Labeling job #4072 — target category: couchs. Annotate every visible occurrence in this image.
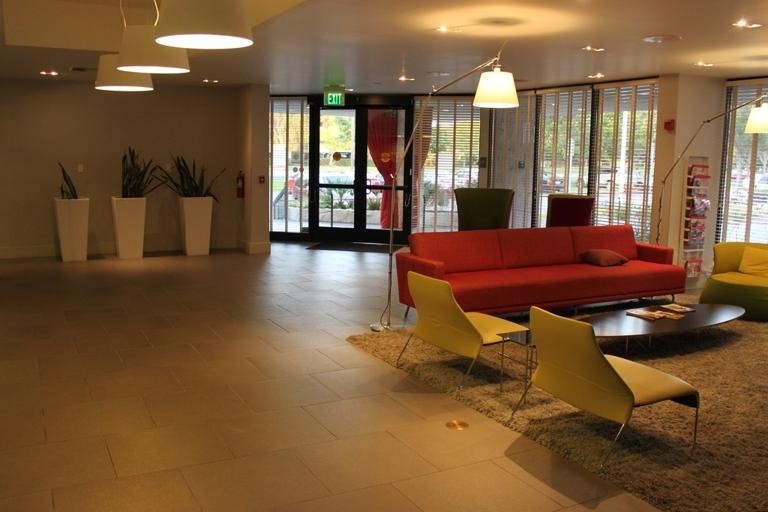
[396,224,686,322]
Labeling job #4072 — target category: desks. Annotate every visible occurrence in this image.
[496,330,535,404]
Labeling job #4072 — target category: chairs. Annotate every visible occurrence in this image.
[396,272,530,401]
[509,305,700,469]
[454,188,515,232]
[699,242,768,323]
[547,194,595,227]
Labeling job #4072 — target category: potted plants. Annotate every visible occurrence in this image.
[150,150,227,256]
[52,161,90,262]
[110,144,168,259]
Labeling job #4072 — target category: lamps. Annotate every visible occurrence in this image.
[370,58,519,332]
[153,0,254,49]
[94,54,154,93]
[656,94,768,246]
[115,24,191,75]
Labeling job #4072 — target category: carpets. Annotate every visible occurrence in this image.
[306,243,404,253]
[346,295,768,512]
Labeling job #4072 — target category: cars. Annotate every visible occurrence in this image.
[286,170,384,203]
[576,165,620,191]
[625,174,653,193]
[540,170,566,192]
[425,168,479,204]
[751,171,768,203]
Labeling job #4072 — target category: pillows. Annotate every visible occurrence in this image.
[738,246,768,277]
[576,249,629,266]
